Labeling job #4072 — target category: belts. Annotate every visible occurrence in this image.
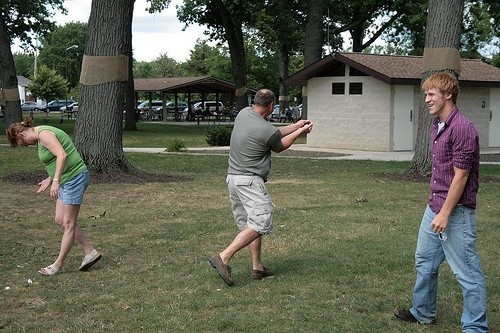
[457,204,463,206]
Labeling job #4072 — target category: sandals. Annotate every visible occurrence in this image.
[79,249,102,272]
[37,264,63,276]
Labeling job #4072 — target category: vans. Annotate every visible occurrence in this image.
[154,101,188,113]
[41,99,75,113]
[182,101,224,117]
[136,100,166,114]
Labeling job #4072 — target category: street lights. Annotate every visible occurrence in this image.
[65,45,78,78]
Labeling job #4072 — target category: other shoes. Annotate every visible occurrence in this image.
[395,309,436,325]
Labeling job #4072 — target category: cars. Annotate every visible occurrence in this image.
[123,100,145,114]
[264,103,303,124]
[59,102,78,113]
[21,101,41,112]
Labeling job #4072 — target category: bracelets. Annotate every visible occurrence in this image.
[53,179,59,181]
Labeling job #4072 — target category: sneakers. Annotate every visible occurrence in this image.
[208,252,234,287]
[251,266,273,280]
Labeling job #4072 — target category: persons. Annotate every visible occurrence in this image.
[395,74,489,333]
[7,117,102,276]
[208,89,314,286]
